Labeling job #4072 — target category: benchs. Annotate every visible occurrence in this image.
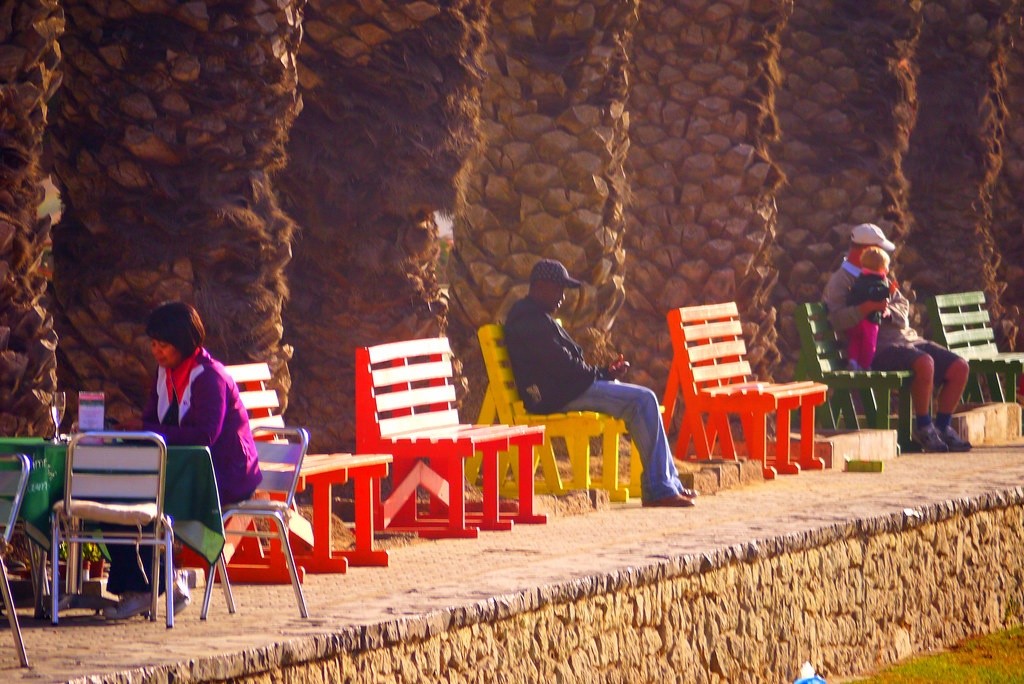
[465,323,665,503]
[173,362,393,586]
[787,301,922,455]
[926,290,1024,435]
[354,337,546,539]
[662,301,828,479]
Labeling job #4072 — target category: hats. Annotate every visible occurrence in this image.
[851,223,896,251]
[531,259,580,288]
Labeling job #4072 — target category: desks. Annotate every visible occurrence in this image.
[0,436,226,621]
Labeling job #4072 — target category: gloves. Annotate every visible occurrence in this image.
[607,354,630,381]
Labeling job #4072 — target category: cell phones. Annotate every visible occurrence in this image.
[617,362,630,371]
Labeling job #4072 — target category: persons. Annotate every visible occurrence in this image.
[826,223,971,454]
[104,303,264,620]
[504,259,698,508]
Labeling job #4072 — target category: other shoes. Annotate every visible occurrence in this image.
[642,495,695,506]
[847,359,873,373]
[682,489,697,498]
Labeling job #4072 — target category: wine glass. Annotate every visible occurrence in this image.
[51,391,66,445]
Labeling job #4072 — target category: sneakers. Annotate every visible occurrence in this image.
[913,425,948,452]
[173,573,191,614]
[940,425,971,450]
[104,593,151,618]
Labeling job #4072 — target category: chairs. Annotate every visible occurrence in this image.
[145,425,310,620]
[51,432,173,628]
[0,453,30,667]
